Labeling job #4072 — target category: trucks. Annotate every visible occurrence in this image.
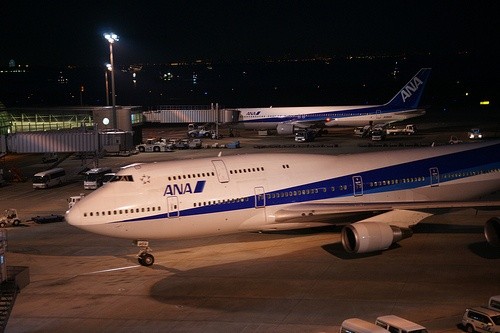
[135,137,176,153]
[354,125,414,141]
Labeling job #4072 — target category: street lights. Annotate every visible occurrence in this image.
[105,64,113,106]
[105,33,120,106]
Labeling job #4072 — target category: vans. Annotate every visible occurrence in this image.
[375,315,428,333]
[340,318,392,333]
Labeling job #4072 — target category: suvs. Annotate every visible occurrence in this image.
[454,295,500,333]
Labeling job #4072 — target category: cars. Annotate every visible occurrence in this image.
[294,132,307,143]
[468,129,484,142]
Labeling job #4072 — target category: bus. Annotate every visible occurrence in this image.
[83,167,112,191]
[32,168,66,190]
[102,173,117,186]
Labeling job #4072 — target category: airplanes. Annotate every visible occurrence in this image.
[234,68,432,143]
[65,140,500,266]
